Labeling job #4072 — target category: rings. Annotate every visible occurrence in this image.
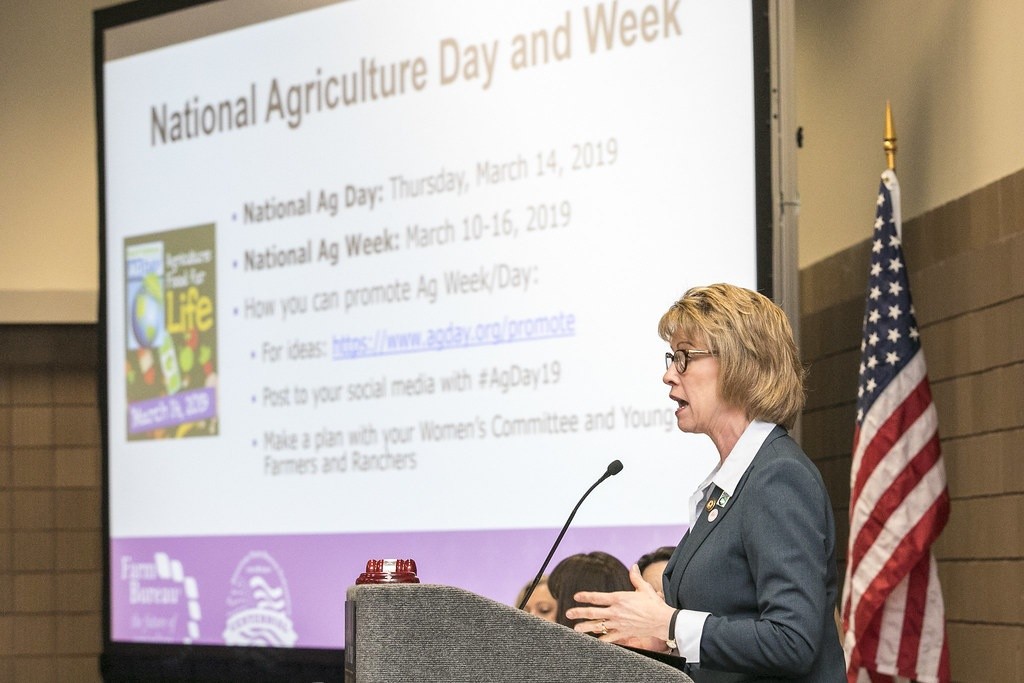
[595,618,611,636]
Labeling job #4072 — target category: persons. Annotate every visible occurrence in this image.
[514,544,679,639]
[566,285,851,683]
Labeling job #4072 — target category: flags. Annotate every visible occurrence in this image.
[832,166,957,683]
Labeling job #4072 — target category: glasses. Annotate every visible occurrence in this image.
[665,350,719,374]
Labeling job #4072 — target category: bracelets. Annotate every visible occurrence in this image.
[664,606,684,657]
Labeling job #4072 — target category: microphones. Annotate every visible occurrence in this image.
[519,460,624,610]
